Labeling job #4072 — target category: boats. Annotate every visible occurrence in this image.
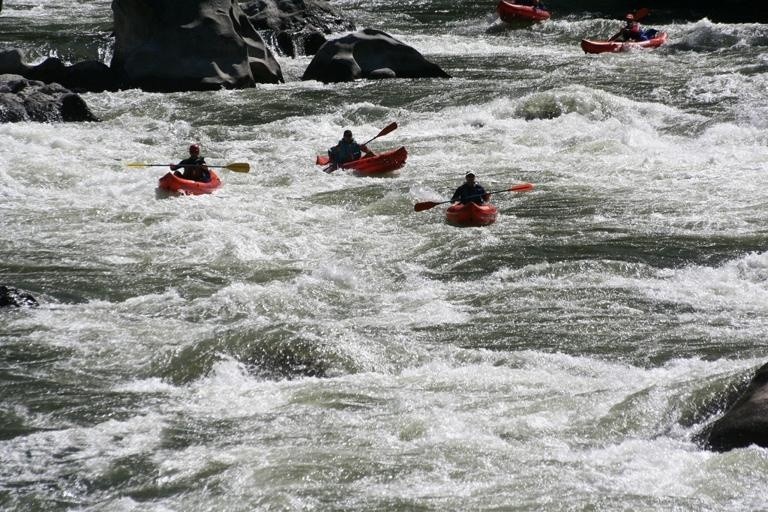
[314,145,409,176]
[447,200,498,228]
[581,32,668,54]
[497,0,551,27]
[157,167,222,196]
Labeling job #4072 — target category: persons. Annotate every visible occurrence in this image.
[618,14,644,43]
[168,144,211,182]
[329,130,377,162]
[450,169,492,207]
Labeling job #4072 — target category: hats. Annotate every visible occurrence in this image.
[466,170,476,177]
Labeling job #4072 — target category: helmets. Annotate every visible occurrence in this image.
[189,144,200,155]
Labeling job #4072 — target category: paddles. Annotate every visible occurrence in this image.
[414,184,532,211]
[127,161,249,172]
[323,122,397,173]
